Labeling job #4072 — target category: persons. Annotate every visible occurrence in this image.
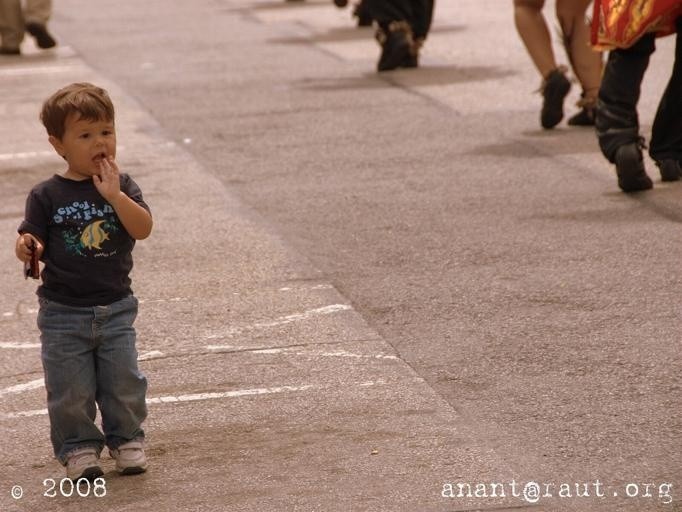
[0,0,57,55]
[593,1,682,192]
[512,1,605,128]
[361,0,436,72]
[15,82,153,483]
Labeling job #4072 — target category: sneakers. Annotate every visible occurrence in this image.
[376,20,421,71]
[108,441,148,474]
[66,448,104,483]
[0,48,20,55]
[657,158,682,182]
[568,109,598,126]
[541,69,570,129]
[25,22,55,49]
[615,144,653,191]
